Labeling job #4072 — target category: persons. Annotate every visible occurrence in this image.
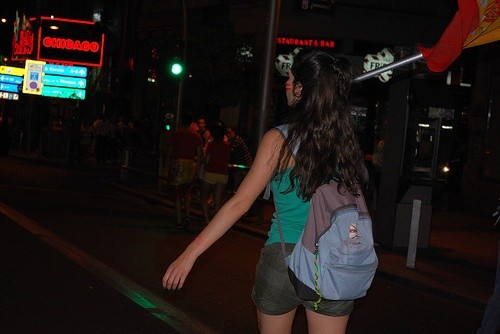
[165,115,255,230]
[162,48,371,334]
[368,139,384,192]
[50,108,152,168]
[405,124,430,188]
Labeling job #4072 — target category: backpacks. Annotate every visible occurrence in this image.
[274,124,379,300]
